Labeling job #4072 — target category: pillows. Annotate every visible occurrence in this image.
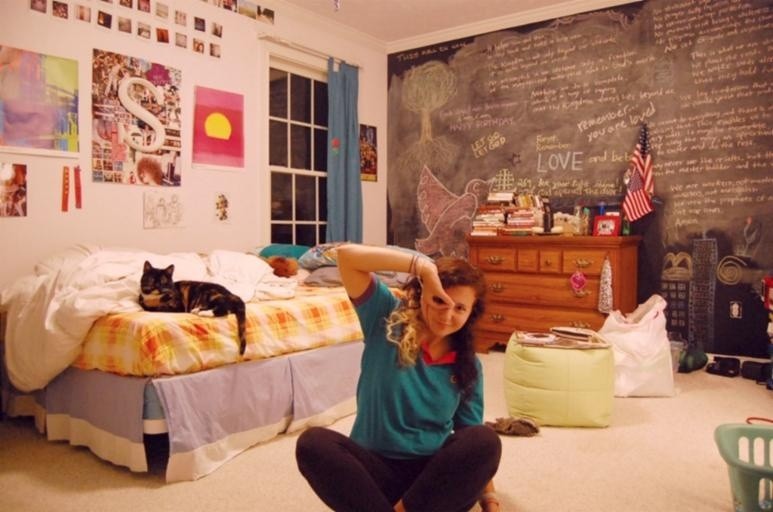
[304,267,395,287]
[252,243,312,262]
[296,241,398,276]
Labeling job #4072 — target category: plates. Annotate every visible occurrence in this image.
[523,332,555,342]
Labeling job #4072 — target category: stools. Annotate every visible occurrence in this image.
[501,324,616,433]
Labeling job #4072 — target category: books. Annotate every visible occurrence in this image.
[471,187,546,237]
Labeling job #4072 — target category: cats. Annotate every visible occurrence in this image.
[137,260,248,356]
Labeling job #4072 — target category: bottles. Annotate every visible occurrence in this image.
[573,206,593,235]
[540,191,552,232]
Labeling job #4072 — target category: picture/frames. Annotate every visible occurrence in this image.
[0,40,85,162]
[603,208,621,216]
[592,214,622,237]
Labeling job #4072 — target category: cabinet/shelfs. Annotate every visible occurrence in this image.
[461,230,643,356]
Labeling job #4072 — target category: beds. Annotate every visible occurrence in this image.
[0,239,437,480]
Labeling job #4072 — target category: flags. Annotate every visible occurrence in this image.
[621,118,655,222]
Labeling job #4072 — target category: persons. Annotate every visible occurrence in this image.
[296,243,501,511]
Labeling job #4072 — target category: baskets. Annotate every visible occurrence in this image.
[712,420,773,512]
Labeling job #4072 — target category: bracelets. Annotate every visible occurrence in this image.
[479,492,499,509]
[408,254,421,275]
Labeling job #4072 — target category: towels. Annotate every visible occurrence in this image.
[596,253,614,314]
